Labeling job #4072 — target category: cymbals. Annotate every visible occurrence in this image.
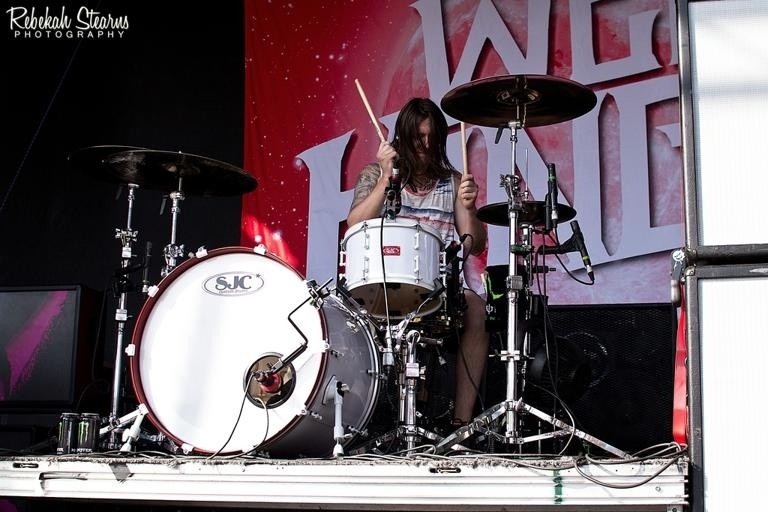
[68,144,257,195]
[442,76,596,128]
[478,201,576,226]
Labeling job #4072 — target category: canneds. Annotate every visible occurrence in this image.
[56,412,98,458]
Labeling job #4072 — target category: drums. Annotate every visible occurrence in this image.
[131,245,383,457]
[55,413,101,456]
[338,218,448,318]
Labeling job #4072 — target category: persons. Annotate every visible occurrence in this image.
[347,97,491,451]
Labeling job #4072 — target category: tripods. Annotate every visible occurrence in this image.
[98,169,179,456]
[345,316,479,456]
[423,119,638,462]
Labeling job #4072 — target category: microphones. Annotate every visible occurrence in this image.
[252,371,282,393]
[548,163,558,221]
[570,220,592,274]
[391,166,402,214]
